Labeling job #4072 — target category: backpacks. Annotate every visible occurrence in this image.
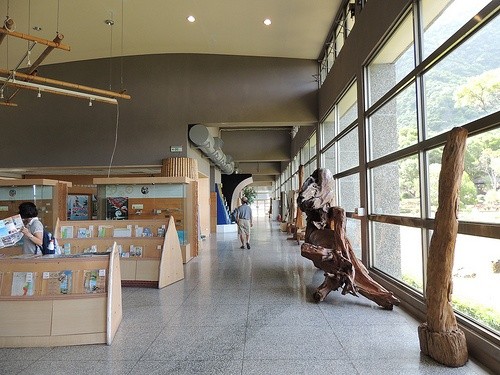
[30,220,56,255]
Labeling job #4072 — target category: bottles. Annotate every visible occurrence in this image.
[49,239,54,250]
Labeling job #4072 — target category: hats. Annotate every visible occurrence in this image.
[242,197,248,202]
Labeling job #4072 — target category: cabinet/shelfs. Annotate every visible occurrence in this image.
[0,178,199,347]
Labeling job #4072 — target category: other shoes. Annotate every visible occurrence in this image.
[240,245,245,249]
[246,243,250,249]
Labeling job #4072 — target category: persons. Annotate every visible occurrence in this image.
[236,197,253,250]
[18,202,55,255]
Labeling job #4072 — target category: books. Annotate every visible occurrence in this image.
[10,224,167,296]
